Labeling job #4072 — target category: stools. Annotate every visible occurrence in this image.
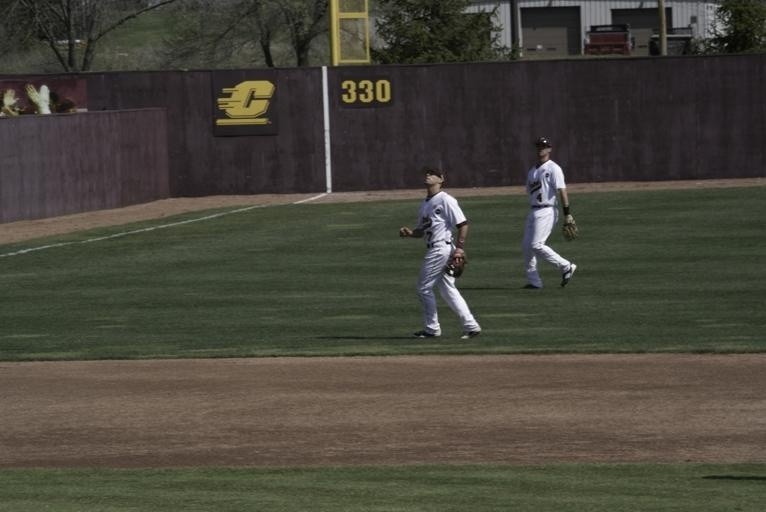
[427,241,450,248]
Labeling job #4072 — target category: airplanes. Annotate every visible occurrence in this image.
[562,213,579,241]
[444,248,466,278]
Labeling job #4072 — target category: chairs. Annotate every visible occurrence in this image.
[560,264,577,287]
[521,284,539,289]
[414,330,440,338]
[460,331,478,339]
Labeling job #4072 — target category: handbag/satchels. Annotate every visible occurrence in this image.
[563,206,570,215]
[456,237,466,249]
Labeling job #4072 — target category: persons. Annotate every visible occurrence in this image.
[1,82,80,117]
[522,136,577,289]
[399,165,482,340]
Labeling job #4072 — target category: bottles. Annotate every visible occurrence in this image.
[422,166,446,179]
[534,136,553,147]
